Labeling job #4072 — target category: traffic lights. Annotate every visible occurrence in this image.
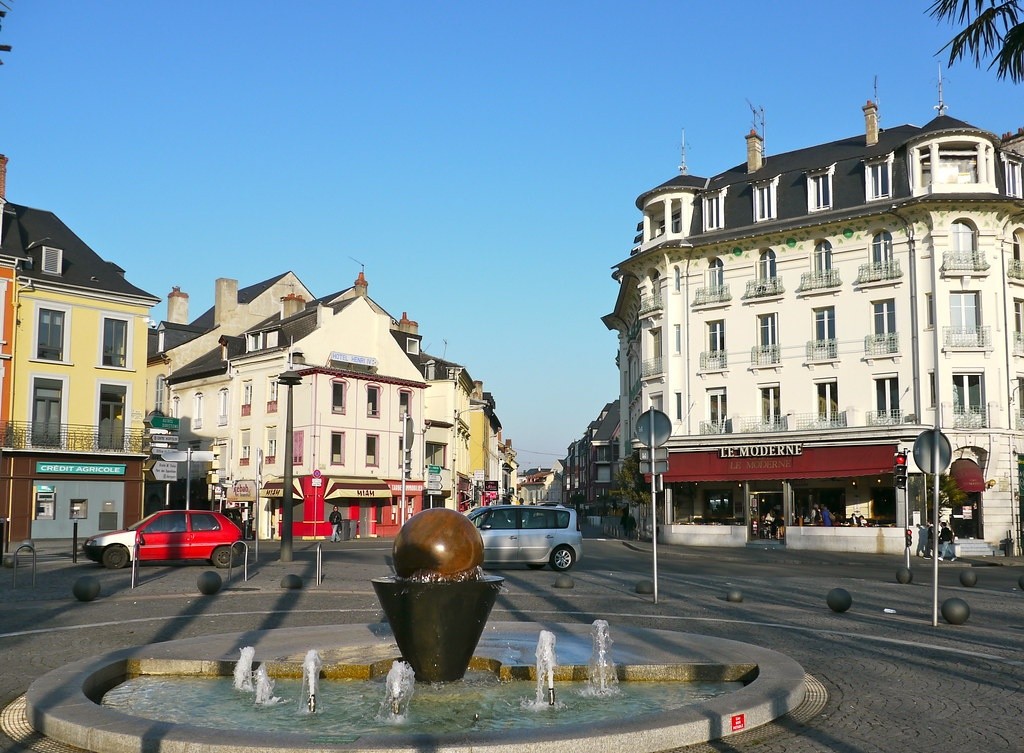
[907,529,912,548]
[894,454,907,489]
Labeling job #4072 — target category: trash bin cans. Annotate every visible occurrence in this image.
[338,519,350,541]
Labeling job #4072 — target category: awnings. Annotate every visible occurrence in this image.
[949,460,986,493]
[324,479,393,500]
[259,478,304,500]
[644,444,898,484]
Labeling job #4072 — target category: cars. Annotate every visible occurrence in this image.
[83,509,242,568]
[466,505,583,570]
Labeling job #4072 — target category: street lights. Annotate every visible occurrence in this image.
[452,404,485,510]
[278,372,302,560]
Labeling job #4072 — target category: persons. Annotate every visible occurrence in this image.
[626,513,636,541]
[765,514,772,539]
[850,513,860,525]
[329,505,342,543]
[622,503,629,516]
[612,503,618,515]
[939,522,957,561]
[813,504,821,523]
[923,519,942,559]
[821,504,831,526]
[620,512,629,536]
[859,515,867,523]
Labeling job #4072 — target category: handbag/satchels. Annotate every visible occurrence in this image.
[829,512,835,521]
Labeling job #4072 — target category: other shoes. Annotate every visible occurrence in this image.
[923,556,931,559]
[951,557,957,561]
[938,556,943,561]
